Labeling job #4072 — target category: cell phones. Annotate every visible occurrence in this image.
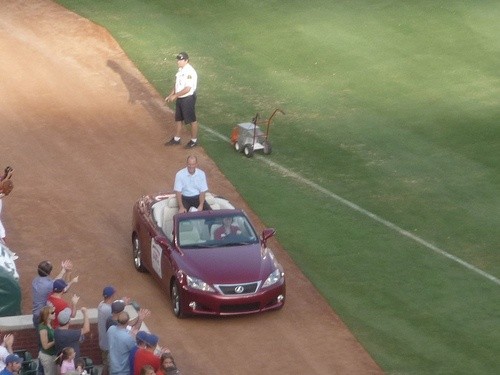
[4,166,13,180]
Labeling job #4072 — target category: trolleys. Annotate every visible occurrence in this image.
[232,107,285,158]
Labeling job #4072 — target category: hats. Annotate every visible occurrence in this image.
[53,279,67,292]
[103,287,116,297]
[57,308,71,324]
[138,333,158,346]
[6,355,20,363]
[112,300,126,312]
[177,52,189,60]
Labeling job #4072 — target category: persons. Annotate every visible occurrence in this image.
[37,306,56,375]
[54,307,89,369]
[32,259,79,375]
[0,329,23,375]
[105,300,180,375]
[214,217,243,242]
[97,286,130,368]
[55,347,83,375]
[174,155,215,235]
[163,51,197,149]
[44,279,80,329]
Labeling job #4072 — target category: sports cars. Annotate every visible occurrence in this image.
[129,192,287,318]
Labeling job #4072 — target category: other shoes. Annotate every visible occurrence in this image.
[165,137,183,145]
[185,140,198,149]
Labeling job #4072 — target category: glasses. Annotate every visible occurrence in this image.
[51,311,55,314]
